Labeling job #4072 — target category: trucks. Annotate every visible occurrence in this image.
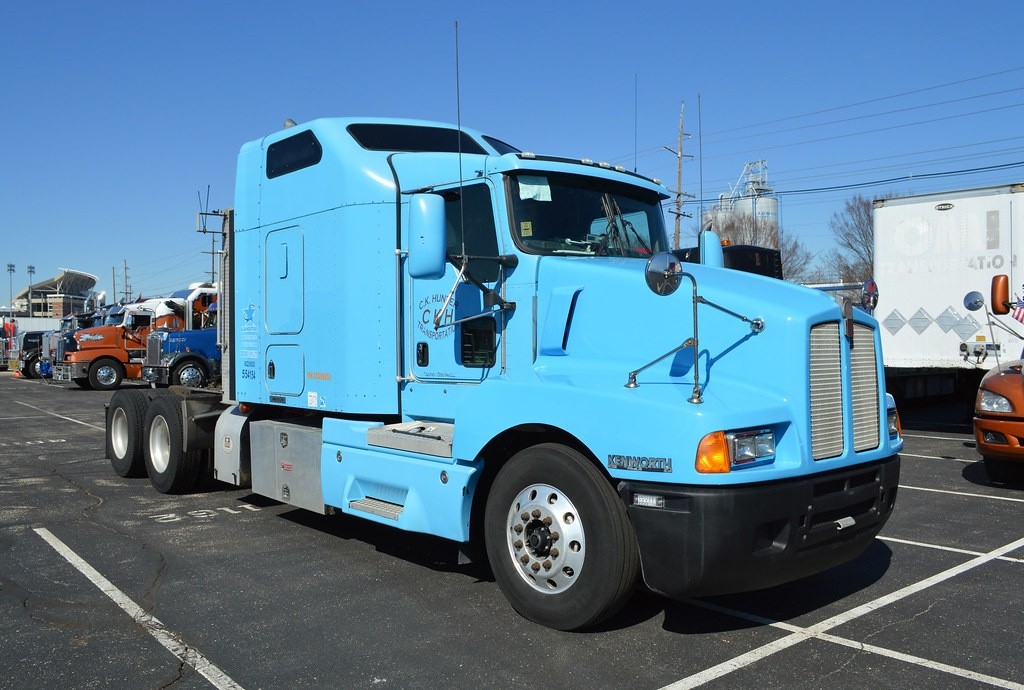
[140,298,224,390]
[871,182,1024,381]
[8,282,220,391]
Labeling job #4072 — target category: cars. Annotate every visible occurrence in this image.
[974,354,1023,481]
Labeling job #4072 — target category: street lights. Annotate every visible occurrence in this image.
[6,263,16,318]
[26,265,36,318]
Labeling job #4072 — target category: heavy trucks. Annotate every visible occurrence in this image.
[101,19,904,631]
